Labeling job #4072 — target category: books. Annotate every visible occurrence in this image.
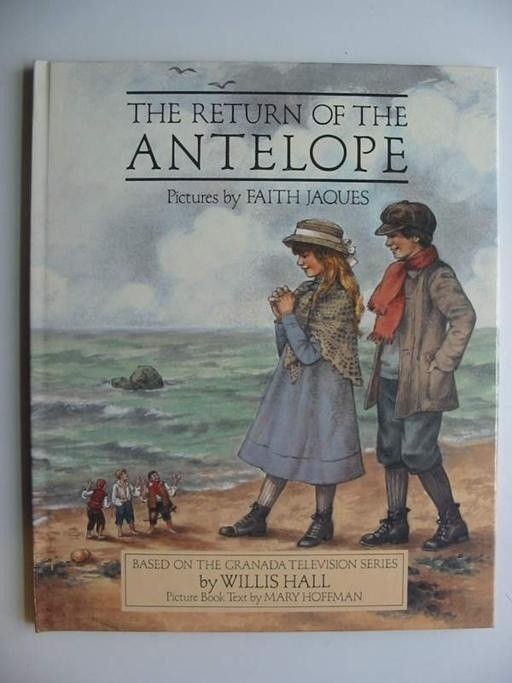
[18,50,503,636]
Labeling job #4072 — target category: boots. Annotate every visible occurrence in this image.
[295,505,336,548]
[218,502,271,538]
[421,504,471,550]
[359,507,411,548]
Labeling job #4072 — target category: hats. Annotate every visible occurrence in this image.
[372,201,439,243]
[281,217,357,261]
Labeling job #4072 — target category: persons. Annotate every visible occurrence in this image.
[217,218,366,550]
[81,477,113,541]
[142,469,182,537]
[110,466,142,538]
[360,199,479,555]
